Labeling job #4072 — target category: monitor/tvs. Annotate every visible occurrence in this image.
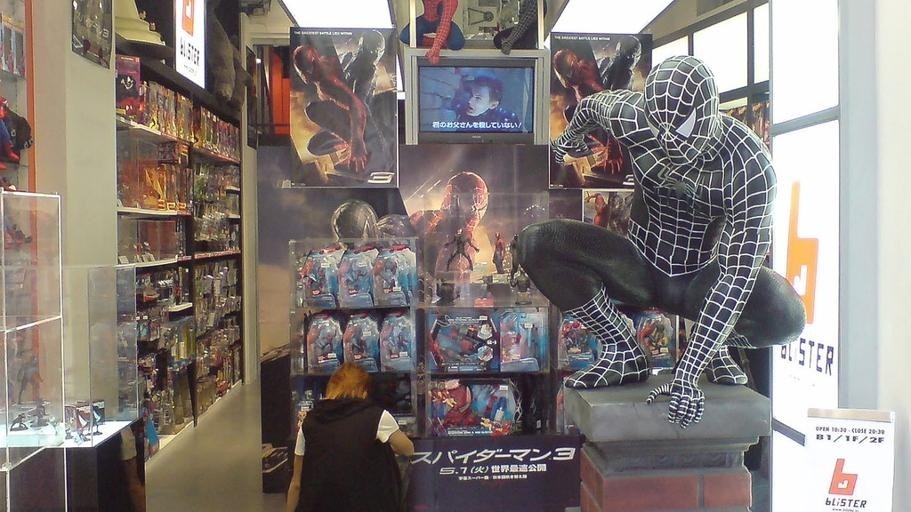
[413,55,536,143]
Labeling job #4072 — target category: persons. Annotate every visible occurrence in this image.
[492,231,506,274]
[595,35,644,93]
[292,44,370,175]
[508,232,525,280]
[510,267,539,306]
[442,228,484,273]
[410,170,491,289]
[334,30,396,176]
[607,192,637,236]
[444,74,522,132]
[330,198,424,262]
[399,0,464,66]
[584,192,610,228]
[553,48,625,178]
[284,362,415,512]
[510,56,806,428]
[0,96,106,444]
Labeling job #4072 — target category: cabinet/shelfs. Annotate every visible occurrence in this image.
[261,37,703,436]
